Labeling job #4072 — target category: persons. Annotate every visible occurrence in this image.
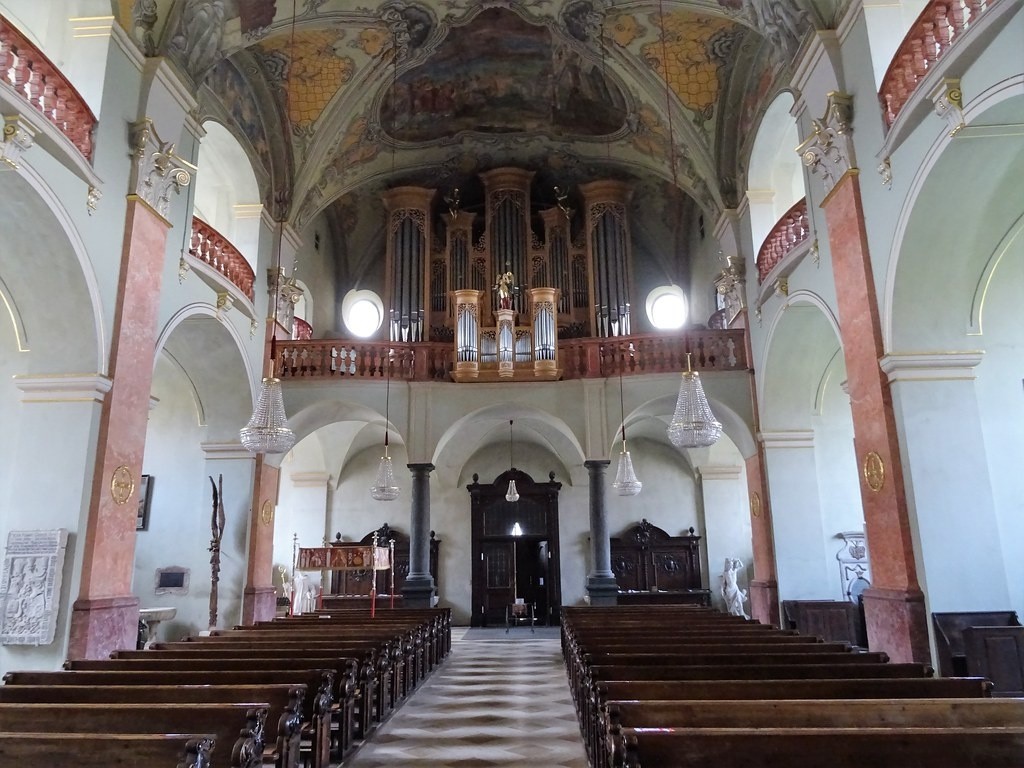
[282,570,318,615]
[719,557,750,622]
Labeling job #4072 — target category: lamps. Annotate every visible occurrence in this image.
[240,1,724,502]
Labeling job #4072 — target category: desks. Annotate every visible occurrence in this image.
[313,592,403,608]
[617,589,713,606]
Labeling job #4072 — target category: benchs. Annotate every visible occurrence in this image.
[0,600,1023,768]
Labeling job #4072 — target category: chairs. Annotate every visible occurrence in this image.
[505,603,537,633]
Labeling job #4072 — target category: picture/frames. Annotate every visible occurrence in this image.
[136,474,149,530]
